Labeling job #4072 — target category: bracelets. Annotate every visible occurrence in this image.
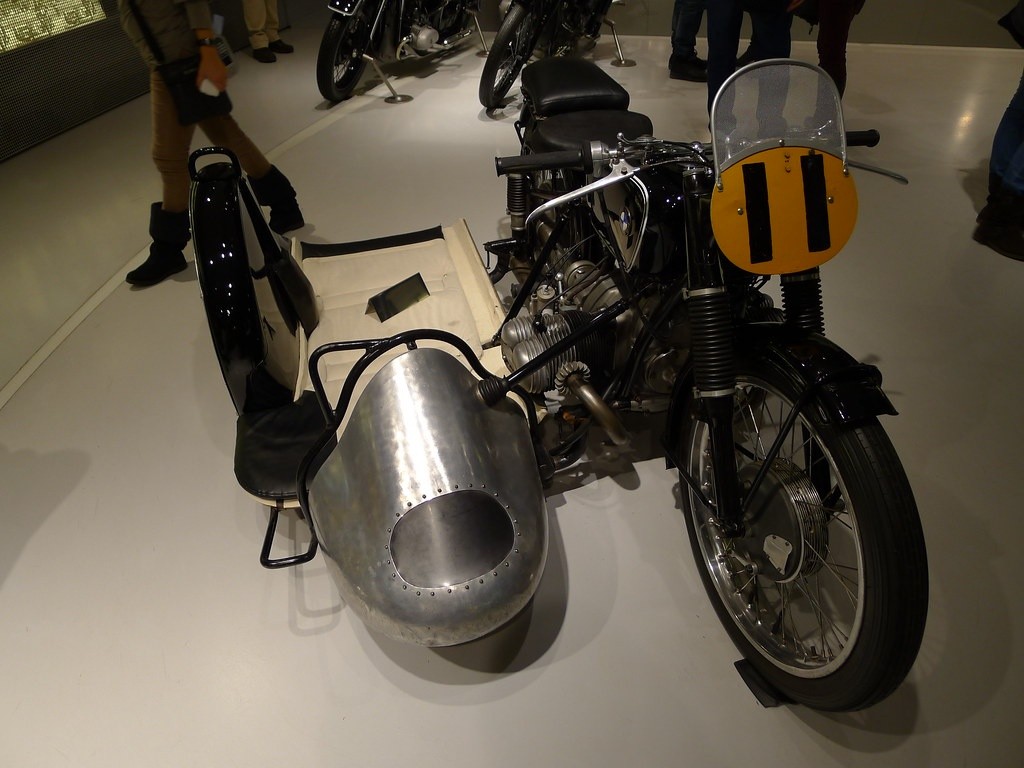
[197,37,214,46]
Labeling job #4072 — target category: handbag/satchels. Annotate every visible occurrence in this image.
[166,55,234,126]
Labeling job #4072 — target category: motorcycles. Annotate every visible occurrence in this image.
[316,0,492,104]
[189,55,930,711]
[479,0,638,108]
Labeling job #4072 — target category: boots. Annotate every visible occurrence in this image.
[125,202,191,288]
[976,172,1004,224]
[972,181,1024,262]
[246,164,305,235]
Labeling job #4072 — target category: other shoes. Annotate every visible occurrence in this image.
[709,115,737,135]
[670,56,708,82]
[252,47,277,64]
[803,116,837,134]
[737,45,757,68]
[668,49,708,70]
[757,117,787,138]
[268,39,294,54]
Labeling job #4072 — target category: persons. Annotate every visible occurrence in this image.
[667,0,864,136]
[124,0,305,288]
[243,0,294,63]
[973,0,1024,262]
